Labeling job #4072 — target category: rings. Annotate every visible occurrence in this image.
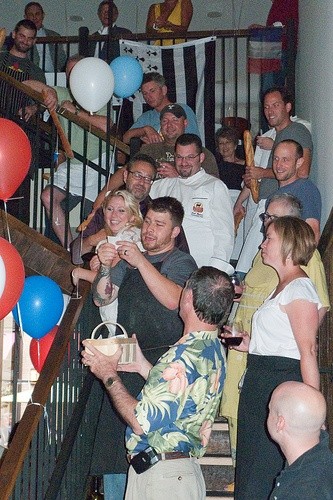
[162,165,166,168]
[124,250,127,255]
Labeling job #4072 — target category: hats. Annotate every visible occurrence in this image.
[160,104,188,118]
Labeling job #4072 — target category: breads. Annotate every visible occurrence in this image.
[42,89,73,158]
[0,27,6,51]
[77,215,92,231]
[244,130,259,203]
[234,206,245,238]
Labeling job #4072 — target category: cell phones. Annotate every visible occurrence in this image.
[142,446,158,465]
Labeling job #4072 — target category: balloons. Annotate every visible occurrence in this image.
[0,118,32,202]
[29,325,59,375]
[0,236,25,320]
[12,275,64,339]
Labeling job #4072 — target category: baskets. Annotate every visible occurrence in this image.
[84,321,136,364]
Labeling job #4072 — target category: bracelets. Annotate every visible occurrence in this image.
[72,269,77,279]
[153,22,159,30]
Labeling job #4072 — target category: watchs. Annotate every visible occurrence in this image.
[103,375,122,390]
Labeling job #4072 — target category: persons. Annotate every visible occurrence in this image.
[0,20,47,227]
[71,71,324,492]
[88,196,197,500]
[81,265,235,500]
[20,55,114,252]
[233,216,322,500]
[71,0,132,66]
[145,0,193,47]
[247,1,299,134]
[23,1,67,72]
[263,380,333,500]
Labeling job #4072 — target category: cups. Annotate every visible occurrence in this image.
[224,320,244,347]
[228,274,243,300]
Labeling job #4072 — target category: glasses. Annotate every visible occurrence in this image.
[259,213,278,222]
[128,170,153,184]
[219,141,233,145]
[175,153,200,161]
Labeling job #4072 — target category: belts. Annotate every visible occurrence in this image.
[127,452,194,464]
